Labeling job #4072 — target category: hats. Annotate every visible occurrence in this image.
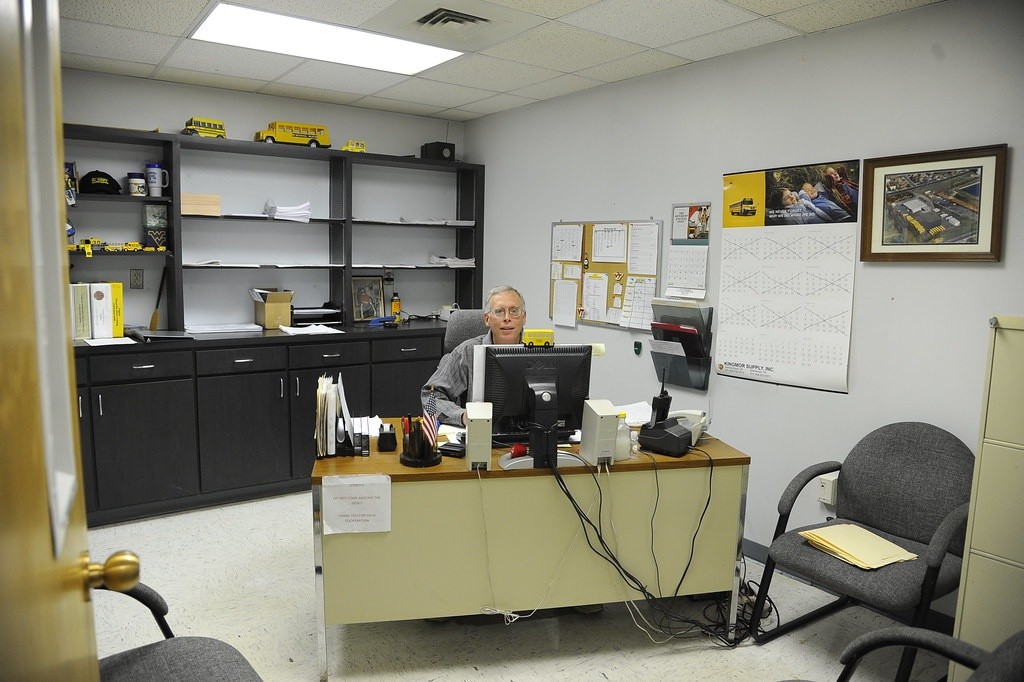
[80,170,123,195]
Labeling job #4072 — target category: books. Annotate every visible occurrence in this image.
[327,384,336,455]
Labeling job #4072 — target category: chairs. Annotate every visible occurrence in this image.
[749,422,975,682]
[779,626,1024,682]
[92,582,265,682]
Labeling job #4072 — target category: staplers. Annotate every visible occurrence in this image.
[369,316,396,327]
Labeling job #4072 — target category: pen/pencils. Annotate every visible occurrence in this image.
[401,413,424,459]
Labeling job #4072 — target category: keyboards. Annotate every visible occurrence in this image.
[457,426,576,443]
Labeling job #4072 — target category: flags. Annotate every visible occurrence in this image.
[421,392,438,447]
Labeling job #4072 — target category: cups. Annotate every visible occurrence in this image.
[128,173,148,196]
[146,164,169,197]
[439,306,450,320]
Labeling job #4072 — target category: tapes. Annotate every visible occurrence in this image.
[383,424,390,432]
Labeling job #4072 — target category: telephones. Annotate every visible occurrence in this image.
[637,368,692,457]
[666,399,712,446]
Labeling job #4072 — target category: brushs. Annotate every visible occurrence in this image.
[148,266,166,331]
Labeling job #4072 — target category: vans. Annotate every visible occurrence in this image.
[521,329,555,348]
[341,140,368,155]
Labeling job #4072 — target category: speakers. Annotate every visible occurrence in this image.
[465,402,493,472]
[579,399,617,467]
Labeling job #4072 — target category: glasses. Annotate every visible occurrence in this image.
[488,308,525,317]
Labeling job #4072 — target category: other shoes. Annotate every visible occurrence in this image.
[574,604,603,614]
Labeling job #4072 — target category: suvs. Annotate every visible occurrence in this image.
[67,237,168,253]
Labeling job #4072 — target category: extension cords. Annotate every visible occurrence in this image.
[737,586,771,619]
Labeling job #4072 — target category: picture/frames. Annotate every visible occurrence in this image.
[860,143,1008,262]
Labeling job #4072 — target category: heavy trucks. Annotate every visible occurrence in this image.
[729,196,760,217]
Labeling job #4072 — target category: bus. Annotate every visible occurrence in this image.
[254,121,332,150]
[180,116,226,140]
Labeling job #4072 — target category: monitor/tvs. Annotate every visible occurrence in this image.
[484,345,593,470]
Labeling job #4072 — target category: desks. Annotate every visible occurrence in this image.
[310,417,751,682]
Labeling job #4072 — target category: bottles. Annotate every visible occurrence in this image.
[630,431,638,459]
[67,219,74,245]
[615,413,629,461]
[391,293,400,318]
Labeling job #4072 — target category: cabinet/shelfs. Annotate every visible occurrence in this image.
[63,122,485,528]
[947,314,1024,682]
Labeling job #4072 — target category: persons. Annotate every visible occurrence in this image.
[774,188,834,225]
[421,286,526,427]
[799,182,854,223]
[822,166,859,217]
